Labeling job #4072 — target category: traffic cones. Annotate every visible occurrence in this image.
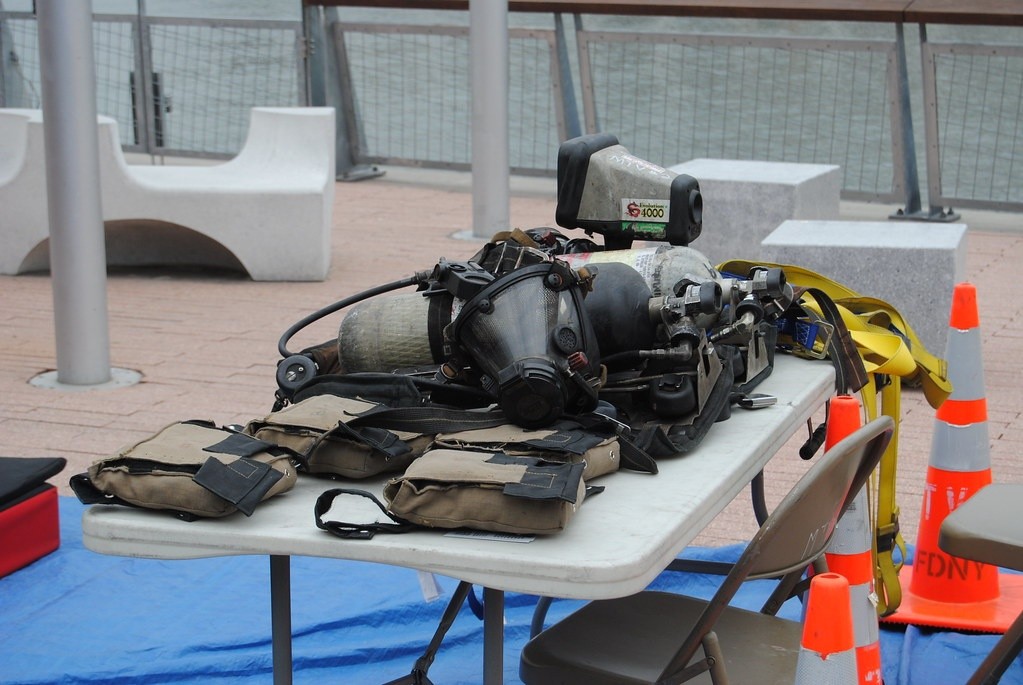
[878,280,1023,634]
[818,395,885,685]
[789,569,859,685]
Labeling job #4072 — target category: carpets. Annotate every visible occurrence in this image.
[0,499,1023,685]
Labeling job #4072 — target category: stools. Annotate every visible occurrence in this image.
[760,219,969,366]
[646,158,842,268]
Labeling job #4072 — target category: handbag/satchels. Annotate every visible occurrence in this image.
[314,448,604,540]
[227,394,439,480]
[68,418,309,524]
[422,404,620,480]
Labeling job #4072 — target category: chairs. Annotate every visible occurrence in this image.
[520,415,896,685]
[938,484,1023,685]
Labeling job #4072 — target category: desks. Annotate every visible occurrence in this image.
[84,349,837,685]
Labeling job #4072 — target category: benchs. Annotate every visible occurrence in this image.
[0,107,337,283]
